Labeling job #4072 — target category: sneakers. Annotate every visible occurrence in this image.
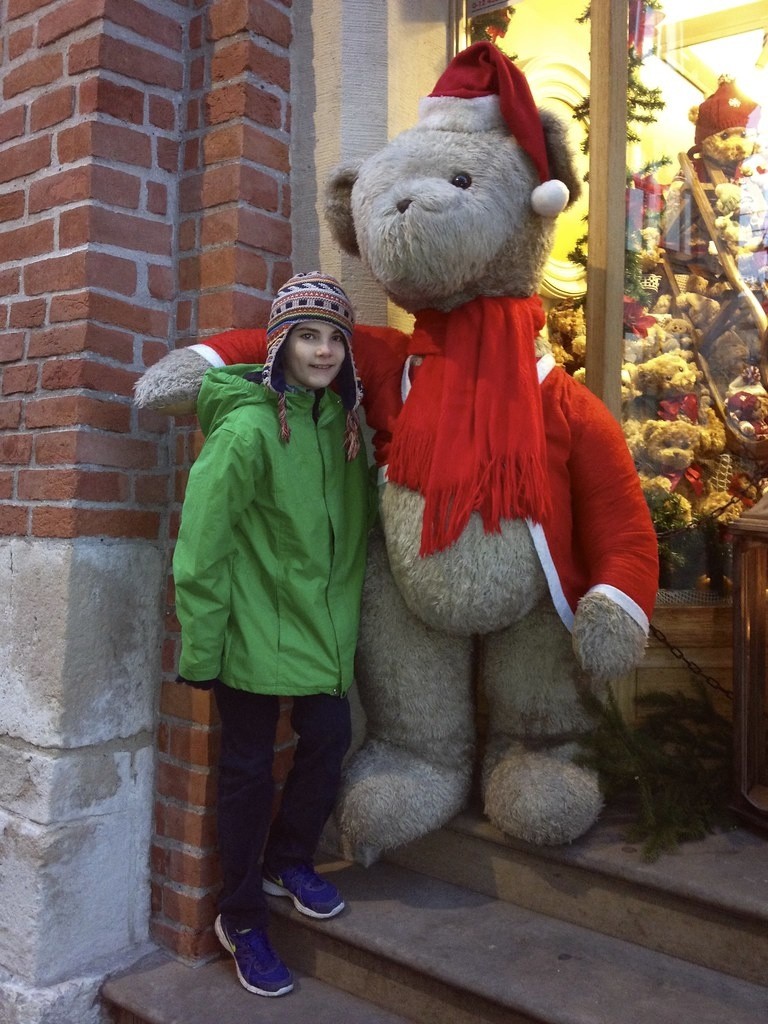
[261,860,345,919]
[214,913,294,997]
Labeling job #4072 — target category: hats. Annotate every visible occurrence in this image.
[261,270,365,461]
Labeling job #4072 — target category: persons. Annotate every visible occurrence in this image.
[172,272,368,994]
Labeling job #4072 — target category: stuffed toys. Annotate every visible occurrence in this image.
[546,74,768,589]
[133,42,660,867]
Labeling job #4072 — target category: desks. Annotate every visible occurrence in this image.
[724,520,768,833]
